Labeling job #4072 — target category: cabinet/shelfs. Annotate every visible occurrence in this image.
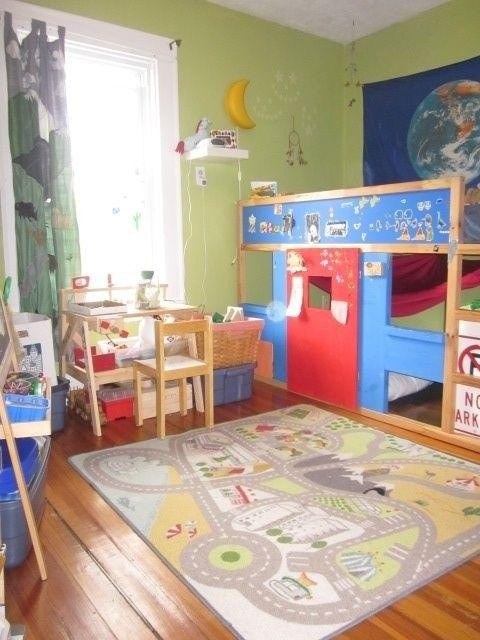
[52,280,208,438]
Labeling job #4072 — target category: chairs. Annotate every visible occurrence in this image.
[128,314,218,444]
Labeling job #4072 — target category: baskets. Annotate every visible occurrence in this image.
[192,310,267,372]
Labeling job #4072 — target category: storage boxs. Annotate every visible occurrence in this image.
[0,393,52,429]
[2,435,39,493]
[190,362,260,408]
[45,374,72,438]
[1,436,57,573]
[8,310,59,383]
[97,388,136,423]
[121,379,194,422]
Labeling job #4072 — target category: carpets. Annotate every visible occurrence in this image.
[66,402,480,640]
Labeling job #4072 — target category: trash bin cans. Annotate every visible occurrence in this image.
[51,376,71,433]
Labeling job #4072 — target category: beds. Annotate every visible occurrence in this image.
[236,173,480,257]
